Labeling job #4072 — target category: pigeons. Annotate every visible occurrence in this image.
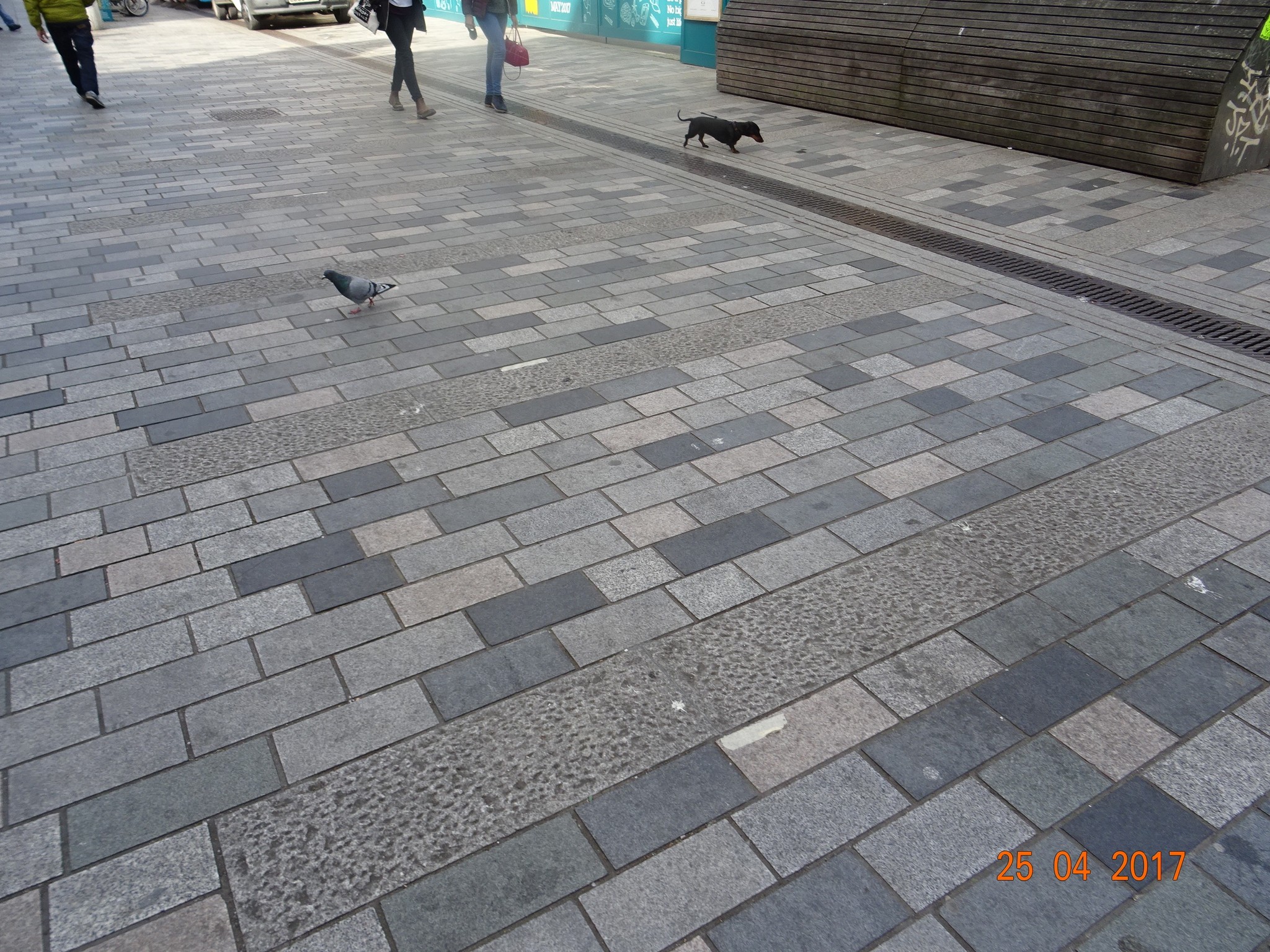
[322,269,398,315]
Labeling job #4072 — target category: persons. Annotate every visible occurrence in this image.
[370,0,437,118]
[0,4,21,31]
[23,0,106,110]
[461,0,518,114]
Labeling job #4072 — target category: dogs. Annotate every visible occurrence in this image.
[677,108,764,154]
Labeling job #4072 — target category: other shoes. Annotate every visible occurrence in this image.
[9,25,21,31]
[80,91,105,108]
[0,27,2,30]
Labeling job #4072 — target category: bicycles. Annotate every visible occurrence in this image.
[108,0,149,17]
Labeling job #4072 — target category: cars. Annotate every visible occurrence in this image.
[158,0,352,30]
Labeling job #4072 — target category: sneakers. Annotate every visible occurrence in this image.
[491,94,508,112]
[484,95,493,108]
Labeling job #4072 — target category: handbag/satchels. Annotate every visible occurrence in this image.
[504,26,529,67]
[347,0,381,34]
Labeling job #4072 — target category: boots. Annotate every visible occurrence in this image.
[415,97,436,118]
[389,90,404,111]
